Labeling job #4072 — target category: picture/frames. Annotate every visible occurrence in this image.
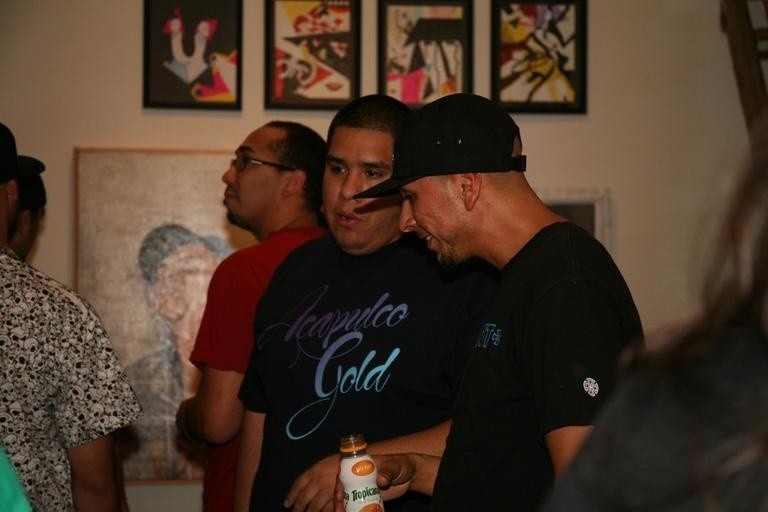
[527,183,609,256]
[376,0,473,113]
[263,0,362,111]
[490,0,587,114]
[143,1,244,111]
[72,146,252,486]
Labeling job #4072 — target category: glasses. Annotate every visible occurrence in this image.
[229,154,293,174]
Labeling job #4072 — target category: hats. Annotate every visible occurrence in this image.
[349,92,530,202]
[0,120,48,187]
[135,220,227,284]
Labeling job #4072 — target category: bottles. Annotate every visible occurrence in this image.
[337,434,384,512]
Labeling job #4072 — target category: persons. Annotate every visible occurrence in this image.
[118,223,225,481]
[176,120,327,511]
[8,156,46,260]
[333,92,646,512]
[544,88,767,511]
[0,123,146,512]
[233,93,499,511]
